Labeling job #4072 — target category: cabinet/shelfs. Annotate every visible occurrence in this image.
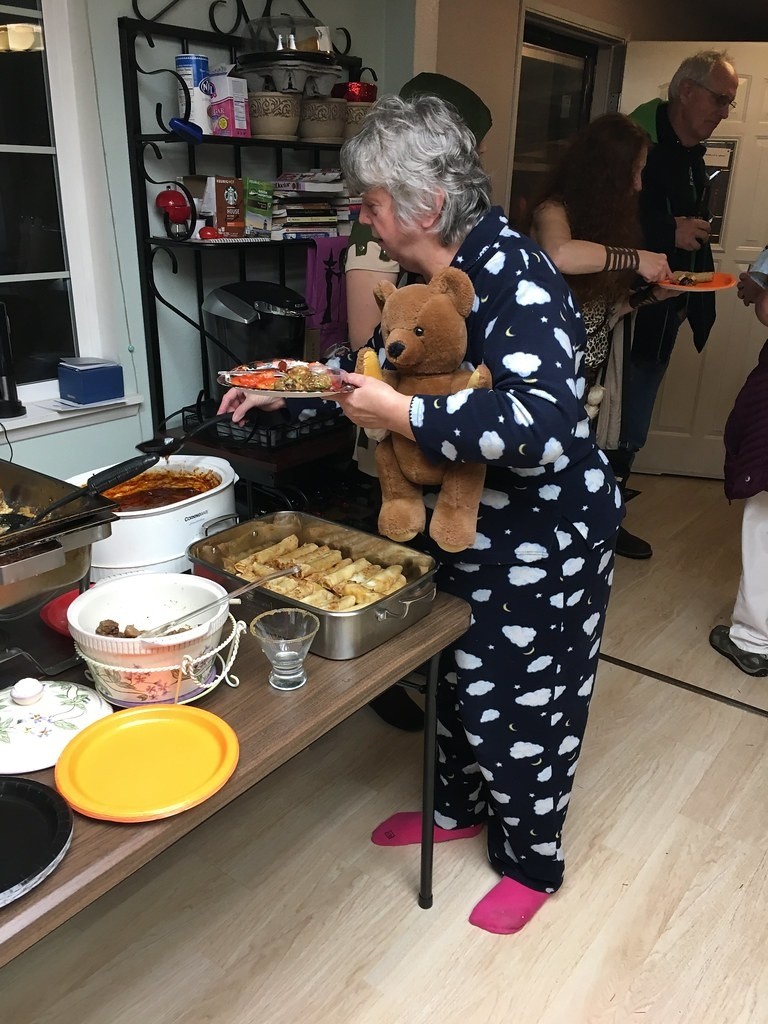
[117,1,378,519]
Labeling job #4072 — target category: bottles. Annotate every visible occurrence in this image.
[688,186,711,249]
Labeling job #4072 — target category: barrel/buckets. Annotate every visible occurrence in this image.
[66,571,231,709]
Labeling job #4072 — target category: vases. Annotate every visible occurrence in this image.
[296,97,351,142]
[344,101,374,142]
[248,88,301,140]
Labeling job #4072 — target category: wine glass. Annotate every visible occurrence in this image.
[249,608,321,692]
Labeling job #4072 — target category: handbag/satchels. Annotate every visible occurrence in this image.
[579,296,610,366]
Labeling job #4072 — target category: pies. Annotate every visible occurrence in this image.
[234,534,406,613]
[670,271,714,282]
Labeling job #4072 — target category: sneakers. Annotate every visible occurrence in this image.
[708,624,768,677]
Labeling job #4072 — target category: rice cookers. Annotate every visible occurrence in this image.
[63,456,240,582]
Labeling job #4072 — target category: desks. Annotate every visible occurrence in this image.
[0,591,474,967]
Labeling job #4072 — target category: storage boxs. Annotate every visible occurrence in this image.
[175,172,244,236]
[56,357,127,405]
[206,64,249,136]
[243,176,274,239]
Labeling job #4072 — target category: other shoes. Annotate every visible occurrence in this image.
[613,526,653,559]
[367,684,426,732]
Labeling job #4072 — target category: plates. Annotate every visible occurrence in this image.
[39,565,225,639]
[54,703,240,824]
[217,358,355,398]
[654,273,736,292]
[0,775,75,909]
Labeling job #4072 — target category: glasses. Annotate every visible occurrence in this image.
[685,77,737,113]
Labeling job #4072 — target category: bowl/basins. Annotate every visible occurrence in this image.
[310,365,348,389]
[245,81,378,138]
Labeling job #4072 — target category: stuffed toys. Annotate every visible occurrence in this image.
[353,266,494,554]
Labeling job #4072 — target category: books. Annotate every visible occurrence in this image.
[214,168,363,239]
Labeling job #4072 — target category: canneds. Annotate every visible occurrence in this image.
[174,55,211,134]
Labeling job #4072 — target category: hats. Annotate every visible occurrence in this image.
[400,71,493,148]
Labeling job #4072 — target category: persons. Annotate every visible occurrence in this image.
[709,244,768,677]
[529,110,685,558]
[343,72,494,734]
[217,94,627,935]
[627,51,738,490]
